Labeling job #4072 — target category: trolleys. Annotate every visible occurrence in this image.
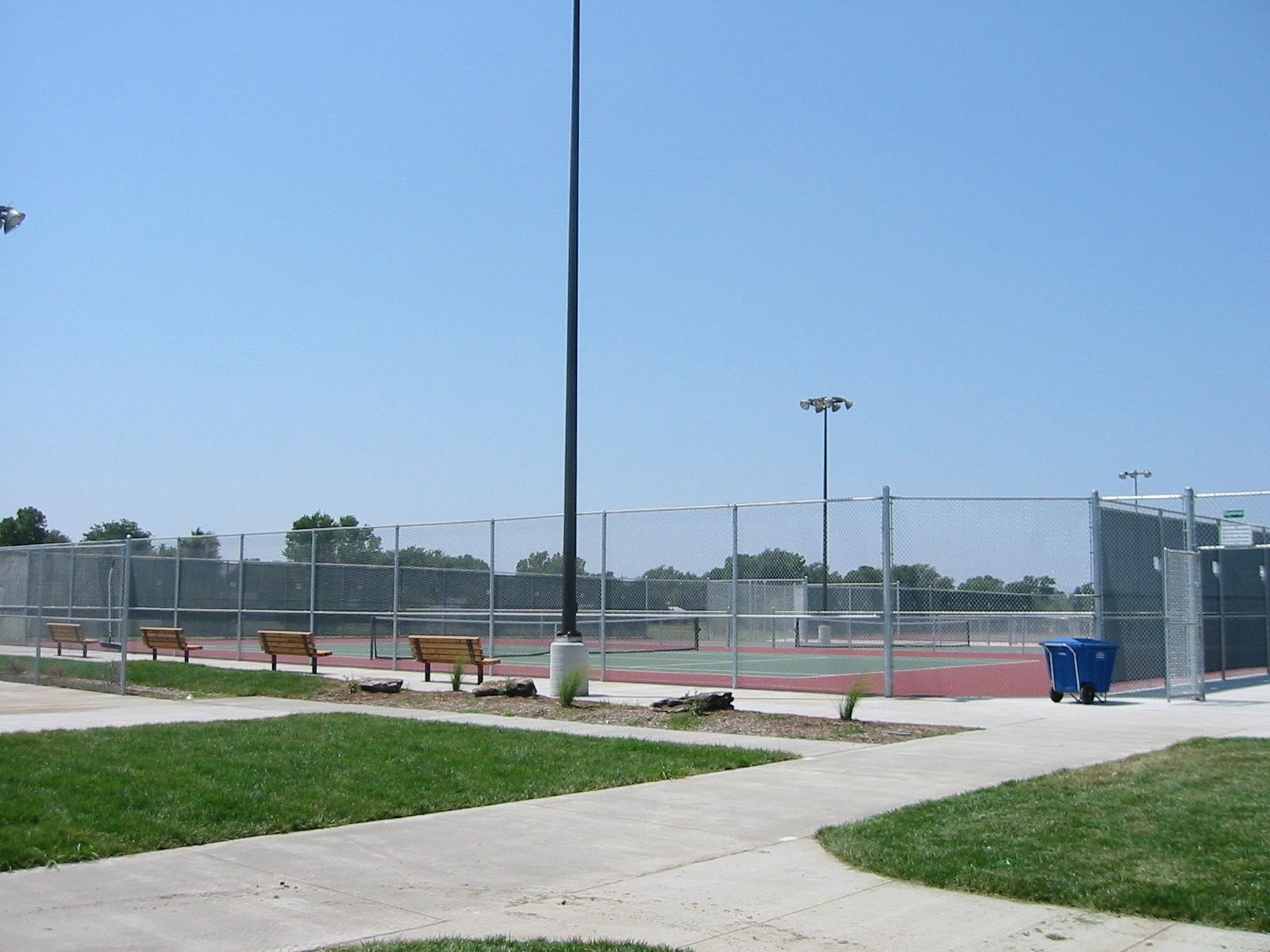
[1039,637,1119,704]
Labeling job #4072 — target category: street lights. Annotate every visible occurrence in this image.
[1120,470,1152,513]
[0,205,25,234]
[799,396,854,643]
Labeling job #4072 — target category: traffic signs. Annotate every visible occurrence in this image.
[1224,510,1244,518]
[1219,524,1253,547]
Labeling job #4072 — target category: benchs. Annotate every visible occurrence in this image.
[45,622,97,658]
[140,626,202,664]
[257,629,334,674]
[406,634,503,687]
